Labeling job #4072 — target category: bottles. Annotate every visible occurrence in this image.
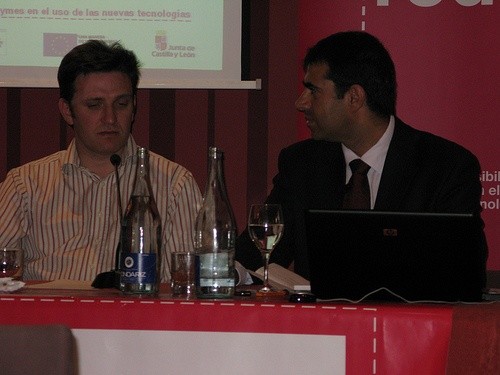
[119,147,163,296]
[195,147,238,299]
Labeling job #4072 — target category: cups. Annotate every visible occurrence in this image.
[0,248,23,280]
[169,252,198,295]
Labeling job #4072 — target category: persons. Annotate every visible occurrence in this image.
[1,41,203,281]
[234,31,488,301]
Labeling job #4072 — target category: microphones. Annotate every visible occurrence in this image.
[90,153,123,289]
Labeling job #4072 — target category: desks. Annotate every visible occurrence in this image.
[0,282,500,375]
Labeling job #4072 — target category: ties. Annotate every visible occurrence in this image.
[346,159,372,210]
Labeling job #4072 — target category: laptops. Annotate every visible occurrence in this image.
[304,209,483,302]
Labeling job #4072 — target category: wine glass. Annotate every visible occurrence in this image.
[248,203,283,295]
